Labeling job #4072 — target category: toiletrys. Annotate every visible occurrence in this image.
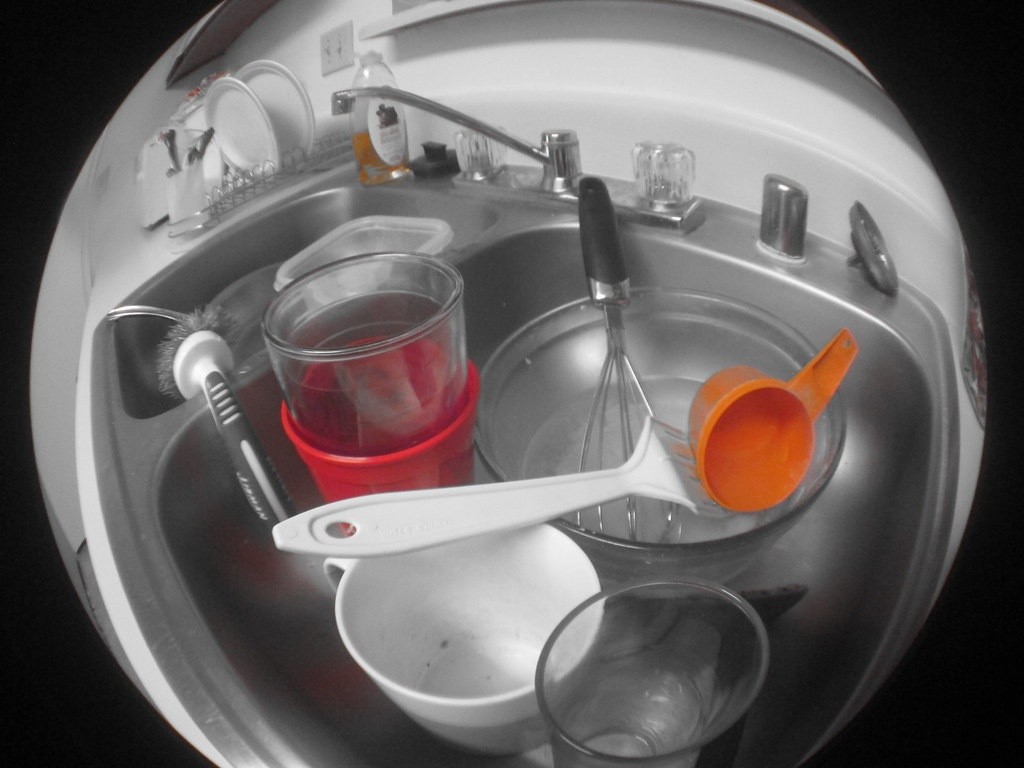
[344,48,410,188]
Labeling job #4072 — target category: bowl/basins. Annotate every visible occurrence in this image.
[213,260,287,327]
[273,214,455,291]
[475,287,848,600]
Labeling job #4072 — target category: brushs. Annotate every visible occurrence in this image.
[153,306,294,536]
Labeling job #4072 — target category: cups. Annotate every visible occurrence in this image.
[279,337,481,537]
[535,578,770,767]
[324,523,604,758]
[261,250,470,456]
[162,159,211,236]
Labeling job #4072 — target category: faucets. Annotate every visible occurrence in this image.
[329,86,581,198]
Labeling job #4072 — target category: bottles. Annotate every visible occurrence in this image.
[350,51,410,186]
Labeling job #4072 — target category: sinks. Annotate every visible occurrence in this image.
[111,182,504,423]
[148,221,935,762]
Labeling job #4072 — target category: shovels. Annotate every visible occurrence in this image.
[269,416,735,559]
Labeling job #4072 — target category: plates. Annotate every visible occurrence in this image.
[206,78,282,181]
[233,59,316,169]
